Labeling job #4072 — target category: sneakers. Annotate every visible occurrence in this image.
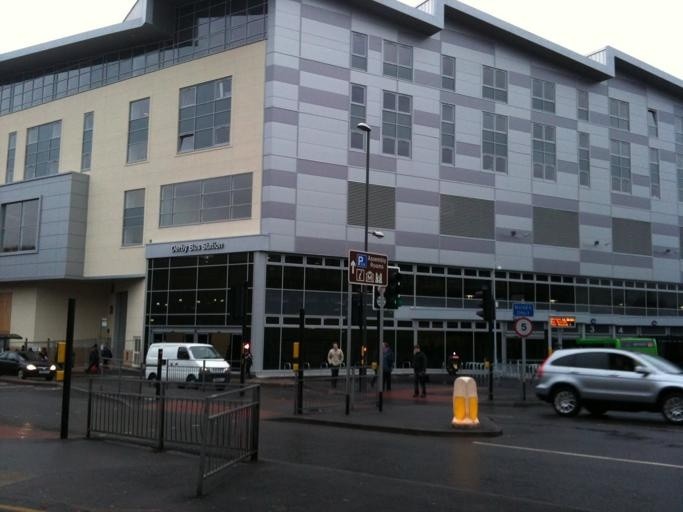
[412,392,427,398]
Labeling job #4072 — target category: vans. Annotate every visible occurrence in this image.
[145,341,230,391]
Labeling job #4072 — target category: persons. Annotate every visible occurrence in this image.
[412,347,427,398]
[3,345,48,360]
[88,344,112,376]
[383,342,394,392]
[242,349,253,377]
[327,342,344,388]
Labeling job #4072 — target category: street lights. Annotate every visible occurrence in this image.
[356,122,383,391]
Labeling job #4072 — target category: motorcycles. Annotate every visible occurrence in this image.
[445,351,459,376]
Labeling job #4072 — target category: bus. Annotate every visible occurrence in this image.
[575,335,683,370]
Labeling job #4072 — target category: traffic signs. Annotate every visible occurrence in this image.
[515,318,532,336]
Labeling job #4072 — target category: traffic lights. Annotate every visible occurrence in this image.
[474,290,489,319]
[242,343,250,355]
[387,269,401,308]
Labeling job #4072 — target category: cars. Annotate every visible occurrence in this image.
[0,348,55,381]
[532,348,683,423]
